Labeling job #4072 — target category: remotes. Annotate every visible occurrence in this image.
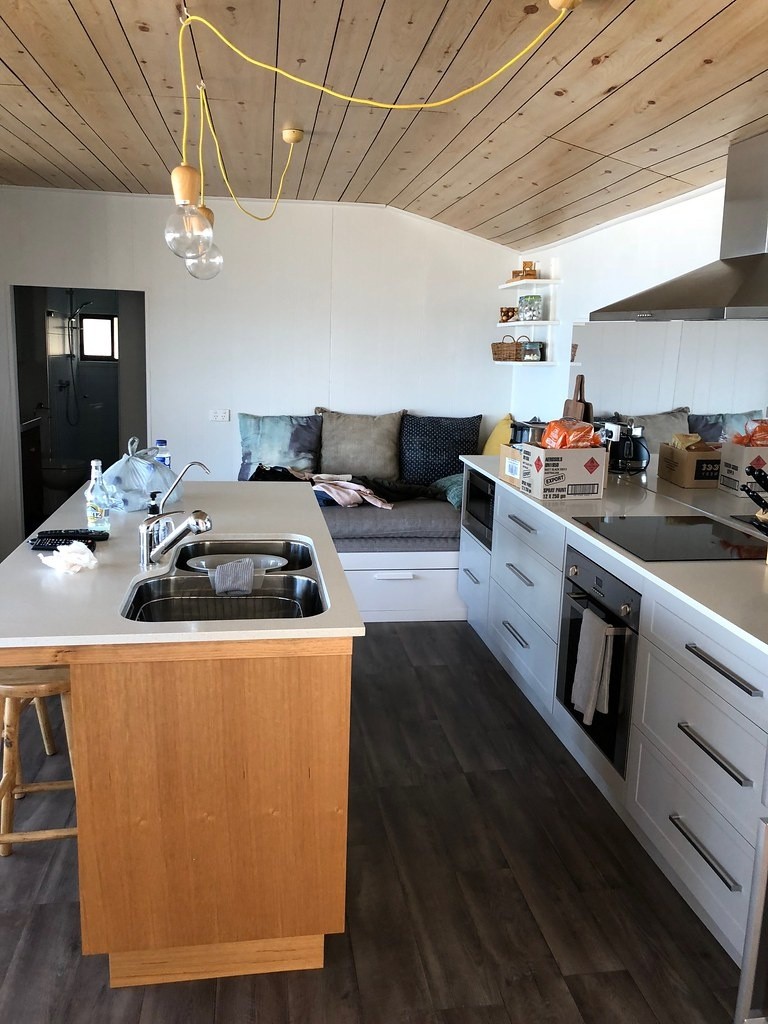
[31,530,109,552]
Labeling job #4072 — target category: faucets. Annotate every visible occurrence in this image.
[159,460,211,515]
[137,509,213,568]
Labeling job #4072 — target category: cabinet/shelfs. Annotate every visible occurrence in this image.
[487,483,567,725]
[0,636,354,988]
[457,527,490,652]
[494,279,556,366]
[625,580,768,970]
[21,426,43,540]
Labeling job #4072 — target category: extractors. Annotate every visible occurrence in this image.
[590,129,768,322]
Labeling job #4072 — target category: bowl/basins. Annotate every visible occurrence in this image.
[186,552,289,575]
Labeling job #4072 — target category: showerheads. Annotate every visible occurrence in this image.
[83,298,96,307]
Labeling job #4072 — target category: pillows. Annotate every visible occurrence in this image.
[399,410,482,486]
[688,414,723,442]
[594,407,690,455]
[428,472,463,511]
[237,413,322,481]
[482,413,513,455]
[723,410,764,438]
[315,407,407,485]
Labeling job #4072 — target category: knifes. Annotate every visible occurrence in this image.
[739,466,768,515]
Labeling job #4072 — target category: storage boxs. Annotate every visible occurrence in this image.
[658,443,721,489]
[719,444,768,498]
[498,442,609,501]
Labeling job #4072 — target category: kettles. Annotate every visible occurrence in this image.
[630,427,649,469]
[608,422,634,474]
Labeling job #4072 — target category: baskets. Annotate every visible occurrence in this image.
[491,335,531,361]
[571,344,579,362]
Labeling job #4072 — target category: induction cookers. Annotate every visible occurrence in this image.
[572,516,768,563]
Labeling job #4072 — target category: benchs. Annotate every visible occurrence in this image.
[322,500,468,622]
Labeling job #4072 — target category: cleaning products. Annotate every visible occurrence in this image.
[147,491,168,549]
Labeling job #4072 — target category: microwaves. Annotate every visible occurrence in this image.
[462,469,496,551]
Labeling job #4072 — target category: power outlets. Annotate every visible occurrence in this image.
[605,420,619,442]
[208,409,229,421]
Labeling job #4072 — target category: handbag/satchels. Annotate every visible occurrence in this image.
[248,462,315,486]
[84,437,183,514]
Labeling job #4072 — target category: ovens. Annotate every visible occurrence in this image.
[556,544,642,780]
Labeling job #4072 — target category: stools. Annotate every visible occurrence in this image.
[0,665,78,857]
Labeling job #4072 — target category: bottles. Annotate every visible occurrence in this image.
[518,294,543,320]
[84,459,111,533]
[154,438,171,469]
[113,463,183,512]
[522,342,541,361]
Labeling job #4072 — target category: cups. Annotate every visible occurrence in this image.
[501,306,518,322]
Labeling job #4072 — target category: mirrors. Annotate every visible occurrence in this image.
[569,320,768,538]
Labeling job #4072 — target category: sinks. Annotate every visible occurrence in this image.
[171,542,317,572]
[124,575,325,621]
